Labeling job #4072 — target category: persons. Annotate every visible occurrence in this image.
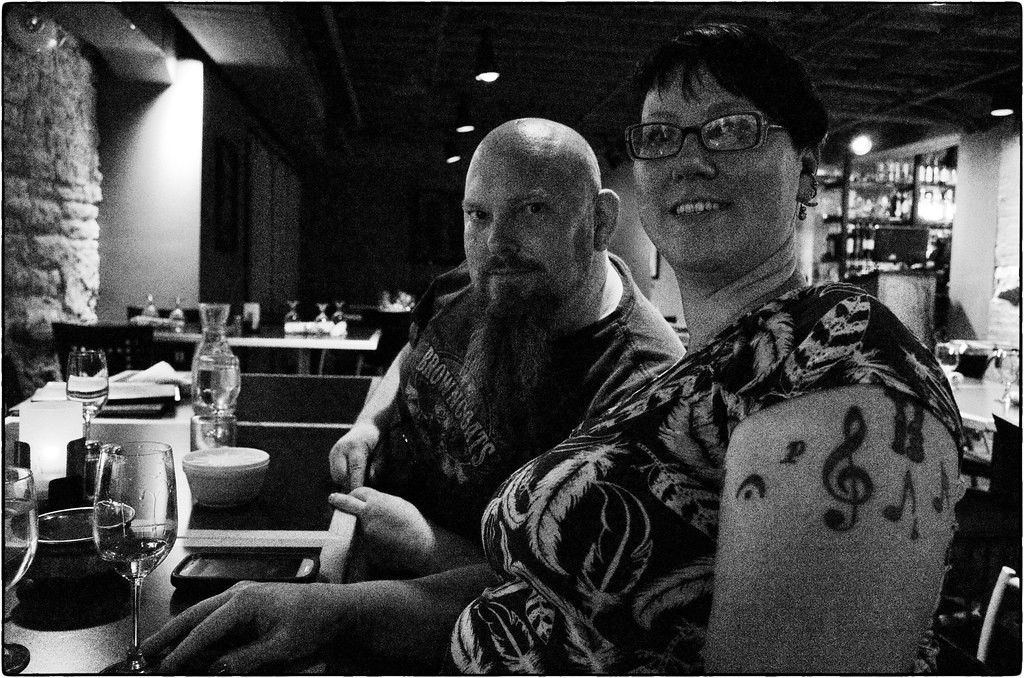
[137,20,966,676]
[328,118,686,581]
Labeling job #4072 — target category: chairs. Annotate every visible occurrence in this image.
[936,413,1022,673]
[49,321,155,382]
[125,305,201,332]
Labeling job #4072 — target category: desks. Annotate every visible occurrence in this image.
[2,303,1022,676]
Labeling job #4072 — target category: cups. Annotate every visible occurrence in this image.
[935,342,960,375]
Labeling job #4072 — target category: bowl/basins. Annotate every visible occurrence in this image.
[955,354,989,381]
[182,447,270,508]
[23,505,136,585]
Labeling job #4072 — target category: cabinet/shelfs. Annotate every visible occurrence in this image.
[815,153,956,280]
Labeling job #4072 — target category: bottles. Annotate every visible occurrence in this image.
[190,416,236,453]
[820,161,956,263]
[192,302,242,418]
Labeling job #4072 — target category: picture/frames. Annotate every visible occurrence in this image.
[213,137,241,256]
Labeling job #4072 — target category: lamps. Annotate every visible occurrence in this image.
[444,32,504,165]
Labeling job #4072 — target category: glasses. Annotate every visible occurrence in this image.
[624,111,786,161]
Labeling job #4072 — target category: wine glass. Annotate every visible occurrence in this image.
[313,303,329,321]
[4,465,39,673]
[332,301,346,324]
[170,296,186,321]
[67,350,110,438]
[91,440,177,677]
[284,301,303,323]
[141,291,159,318]
[994,350,1019,419]
